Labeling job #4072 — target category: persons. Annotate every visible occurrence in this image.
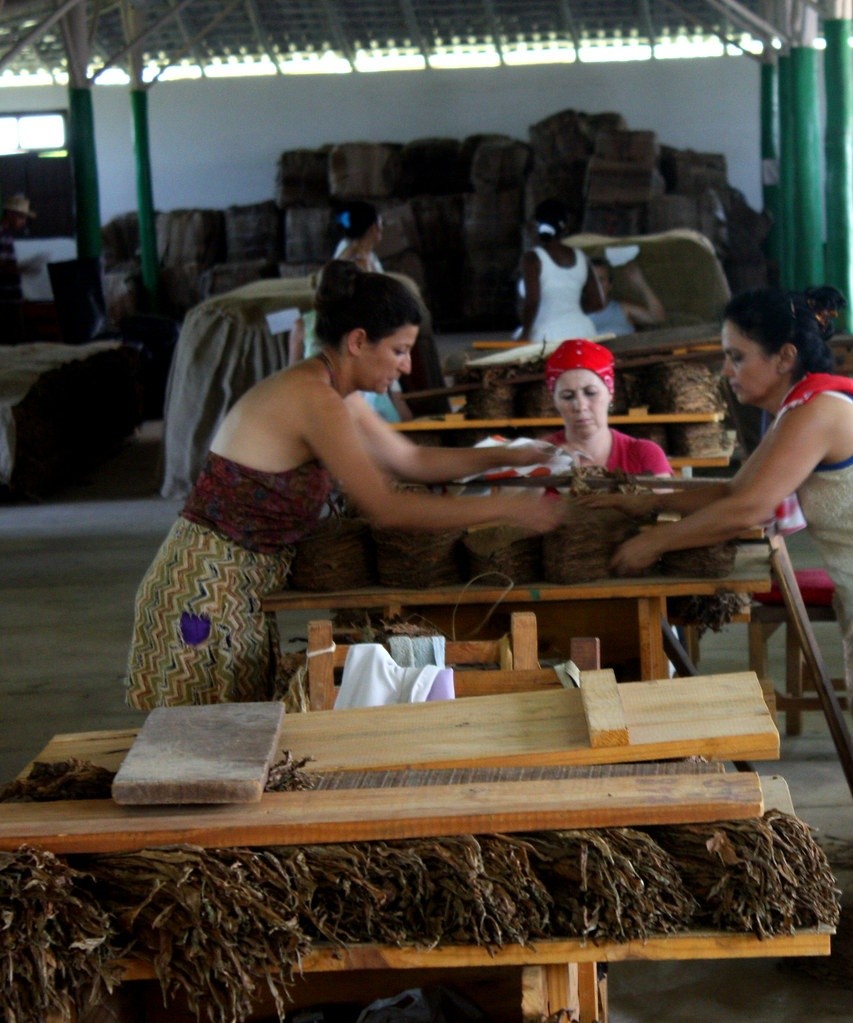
[0,194,37,234]
[524,197,597,343]
[539,336,674,493]
[288,204,413,424]
[587,258,665,336]
[124,258,566,712]
[611,286,853,712]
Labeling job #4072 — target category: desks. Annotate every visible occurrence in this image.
[667,457,729,478]
[261,554,770,682]
[105,928,830,1023]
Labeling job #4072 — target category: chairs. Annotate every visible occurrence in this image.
[306,612,537,712]
[348,637,600,708]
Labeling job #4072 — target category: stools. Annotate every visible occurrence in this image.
[746,570,847,738]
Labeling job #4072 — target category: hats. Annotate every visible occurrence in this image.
[543,338,616,394]
[1,193,36,219]
